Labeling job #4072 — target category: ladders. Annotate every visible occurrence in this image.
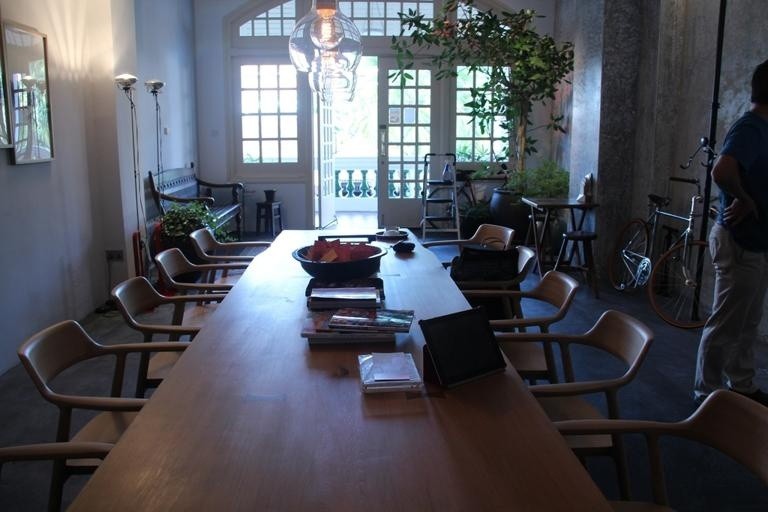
[422,153,461,242]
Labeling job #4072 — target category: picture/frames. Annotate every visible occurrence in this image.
[0,17,56,166]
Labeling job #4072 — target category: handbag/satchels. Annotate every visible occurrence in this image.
[452,236,519,299]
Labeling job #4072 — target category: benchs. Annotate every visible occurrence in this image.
[148,161,244,241]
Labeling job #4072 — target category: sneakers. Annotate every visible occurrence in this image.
[731,386,767,407]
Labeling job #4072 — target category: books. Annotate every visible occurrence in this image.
[305,282,391,310]
[356,353,425,397]
[299,306,417,348]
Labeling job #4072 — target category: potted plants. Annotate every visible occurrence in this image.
[388,2,574,243]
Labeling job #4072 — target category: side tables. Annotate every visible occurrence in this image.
[256,200,283,237]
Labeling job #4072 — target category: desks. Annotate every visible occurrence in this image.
[78,228,601,512]
[521,197,600,283]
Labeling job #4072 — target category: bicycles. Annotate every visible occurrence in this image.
[423,164,508,233]
[606,175,721,330]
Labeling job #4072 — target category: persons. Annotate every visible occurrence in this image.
[691,62,764,413]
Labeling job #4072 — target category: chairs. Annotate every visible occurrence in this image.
[190,227,271,305]
[453,244,537,333]
[551,389,767,511]
[18,318,194,510]
[493,309,656,501]
[459,270,580,384]
[111,276,227,399]
[422,223,516,269]
[156,247,250,341]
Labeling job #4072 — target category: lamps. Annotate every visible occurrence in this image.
[287,0,364,73]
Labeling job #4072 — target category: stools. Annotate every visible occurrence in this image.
[554,231,600,299]
[524,214,556,262]
